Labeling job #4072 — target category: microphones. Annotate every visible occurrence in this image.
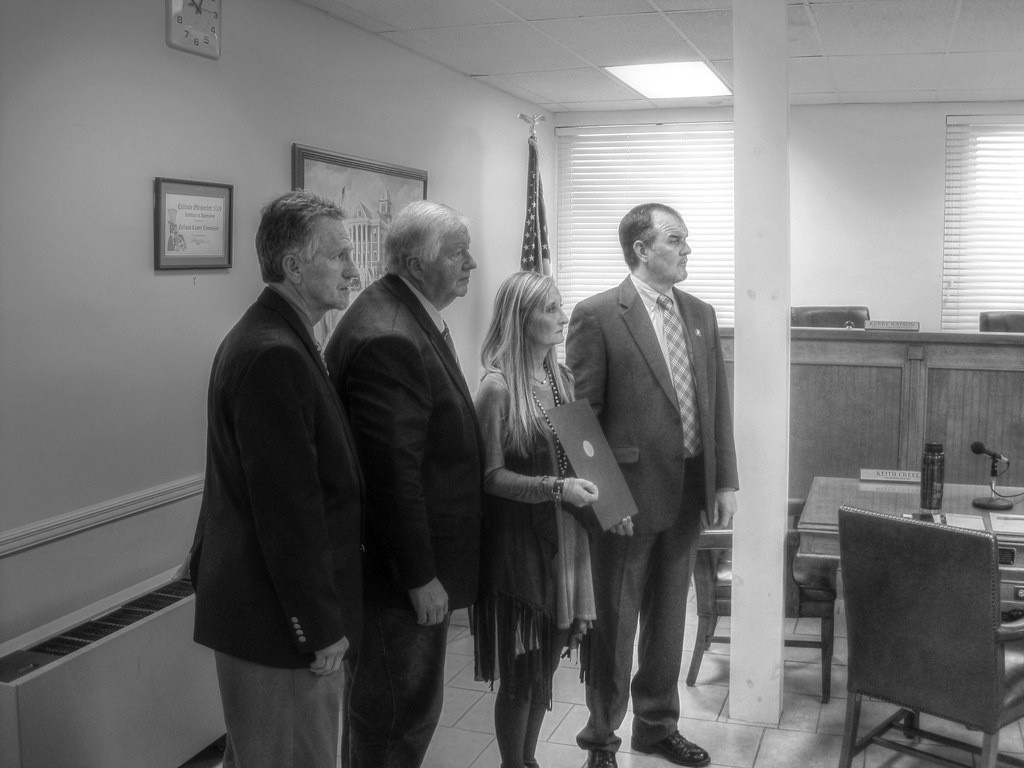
[970,441,1009,464]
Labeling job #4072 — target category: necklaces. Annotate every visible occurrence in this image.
[532,374,548,384]
[531,361,567,477]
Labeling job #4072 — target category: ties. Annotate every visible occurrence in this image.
[315,341,330,375]
[656,294,702,456]
[440,320,462,372]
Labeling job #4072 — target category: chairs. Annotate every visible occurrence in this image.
[790,306,870,328]
[836,502,1024,768]
[686,499,839,704]
[979,310,1024,333]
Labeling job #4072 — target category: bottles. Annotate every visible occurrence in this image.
[920,443,945,509]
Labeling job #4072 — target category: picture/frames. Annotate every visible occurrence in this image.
[153,176,235,271]
[291,142,429,345]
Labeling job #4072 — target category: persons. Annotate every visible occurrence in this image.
[467,273,633,768]
[320,198,484,768]
[189,186,388,768]
[565,203,743,768]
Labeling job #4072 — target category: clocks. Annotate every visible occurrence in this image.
[164,0,223,60]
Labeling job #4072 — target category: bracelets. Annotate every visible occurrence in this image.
[550,477,565,505]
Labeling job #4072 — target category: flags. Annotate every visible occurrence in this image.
[519,137,555,281]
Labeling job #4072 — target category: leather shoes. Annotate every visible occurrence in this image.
[588,749,618,768]
[631,730,711,767]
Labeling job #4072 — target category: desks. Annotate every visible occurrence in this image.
[717,326,1024,500]
[795,475,1023,740]
